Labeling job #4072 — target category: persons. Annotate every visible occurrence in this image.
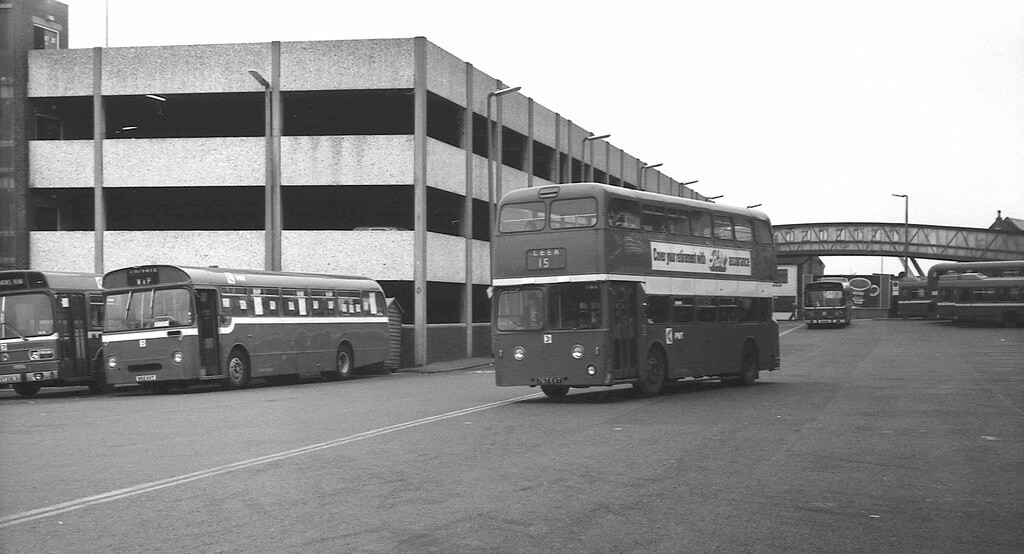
[614,214,630,227]
[518,290,544,329]
[788,301,799,321]
[815,300,821,307]
[665,220,675,233]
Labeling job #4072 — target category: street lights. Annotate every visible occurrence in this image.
[487,87,521,241]
[582,134,611,182]
[892,194,908,277]
[706,196,723,202]
[679,181,698,197]
[640,164,663,190]
[248,69,272,272]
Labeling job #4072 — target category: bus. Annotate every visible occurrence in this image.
[101,265,390,392]
[936,273,1024,328]
[927,261,1024,313]
[0,270,119,396]
[491,182,780,397]
[897,276,1024,317]
[803,278,852,329]
